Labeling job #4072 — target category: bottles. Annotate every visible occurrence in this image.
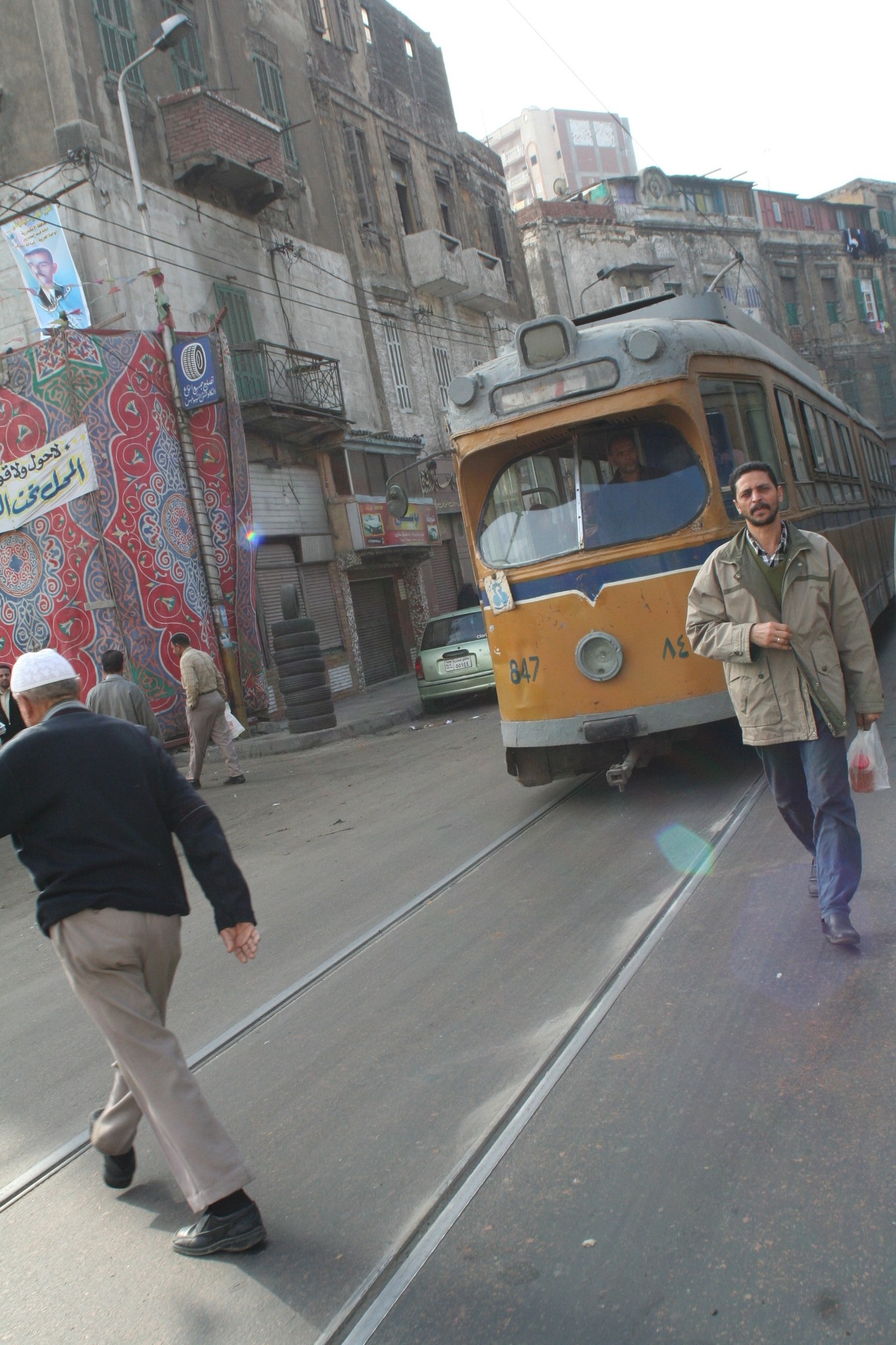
[849,754,875,793]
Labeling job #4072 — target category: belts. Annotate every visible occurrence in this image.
[198,689,216,696]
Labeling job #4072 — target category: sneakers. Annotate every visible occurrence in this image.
[223,774,246,784]
[188,779,201,789]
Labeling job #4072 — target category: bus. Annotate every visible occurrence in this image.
[382,247,895,788]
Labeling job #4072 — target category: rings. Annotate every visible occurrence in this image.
[777,638,779,643]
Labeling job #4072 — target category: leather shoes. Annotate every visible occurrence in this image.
[171,1201,268,1257]
[90,1108,136,1188]
[821,914,860,944]
[808,857,819,897]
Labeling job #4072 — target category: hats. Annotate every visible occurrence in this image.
[10,648,77,692]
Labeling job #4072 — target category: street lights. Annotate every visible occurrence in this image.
[115,11,250,740]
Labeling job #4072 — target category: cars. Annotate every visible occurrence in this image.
[416,604,497,712]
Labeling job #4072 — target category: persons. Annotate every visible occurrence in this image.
[24,248,65,307]
[687,459,885,945]
[457,582,480,610]
[86,649,164,747]
[0,649,268,1256]
[519,429,757,560]
[170,633,245,791]
[0,665,27,747]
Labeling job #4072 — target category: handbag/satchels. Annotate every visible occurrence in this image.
[847,722,890,792]
[224,702,245,739]
